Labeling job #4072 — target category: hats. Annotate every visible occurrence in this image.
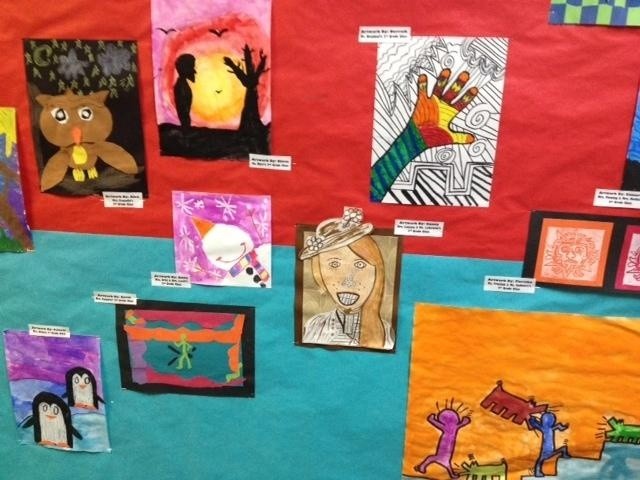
[298,219,373,262]
